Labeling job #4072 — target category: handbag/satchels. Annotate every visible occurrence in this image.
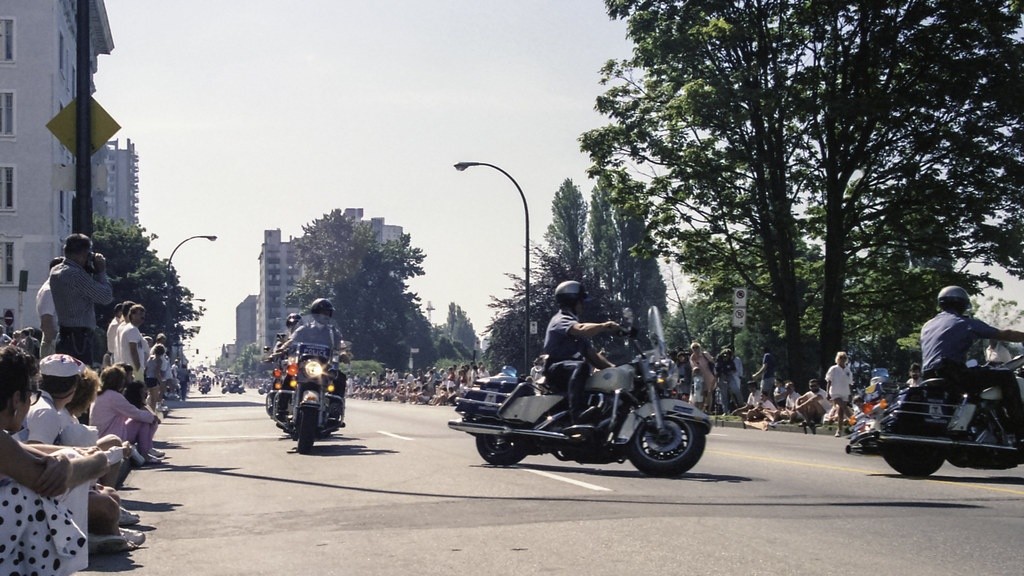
[708,361,718,375]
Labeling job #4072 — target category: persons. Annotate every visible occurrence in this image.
[270,298,350,428]
[234,376,239,383]
[674,342,863,437]
[906,363,922,387]
[534,357,543,380]
[542,281,627,424]
[202,375,211,384]
[920,286,1024,439]
[0,234,190,576]
[986,339,1012,363]
[346,361,490,406]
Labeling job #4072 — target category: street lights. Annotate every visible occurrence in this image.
[166,235,219,357]
[452,161,532,378]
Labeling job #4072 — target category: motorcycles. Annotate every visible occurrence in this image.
[257,333,355,455]
[445,305,713,478]
[845,339,1024,476]
[198,376,246,396]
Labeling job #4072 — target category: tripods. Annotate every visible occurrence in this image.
[712,363,747,429]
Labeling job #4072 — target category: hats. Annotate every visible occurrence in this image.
[37,353,89,380]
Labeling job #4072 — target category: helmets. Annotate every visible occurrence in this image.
[285,313,303,329]
[937,286,973,312]
[554,281,589,302]
[310,299,336,323]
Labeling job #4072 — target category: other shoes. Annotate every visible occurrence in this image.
[835,430,841,437]
[148,447,166,458]
[118,508,144,527]
[798,421,809,428]
[119,527,147,546]
[143,454,164,466]
[574,405,602,426]
[83,532,128,556]
